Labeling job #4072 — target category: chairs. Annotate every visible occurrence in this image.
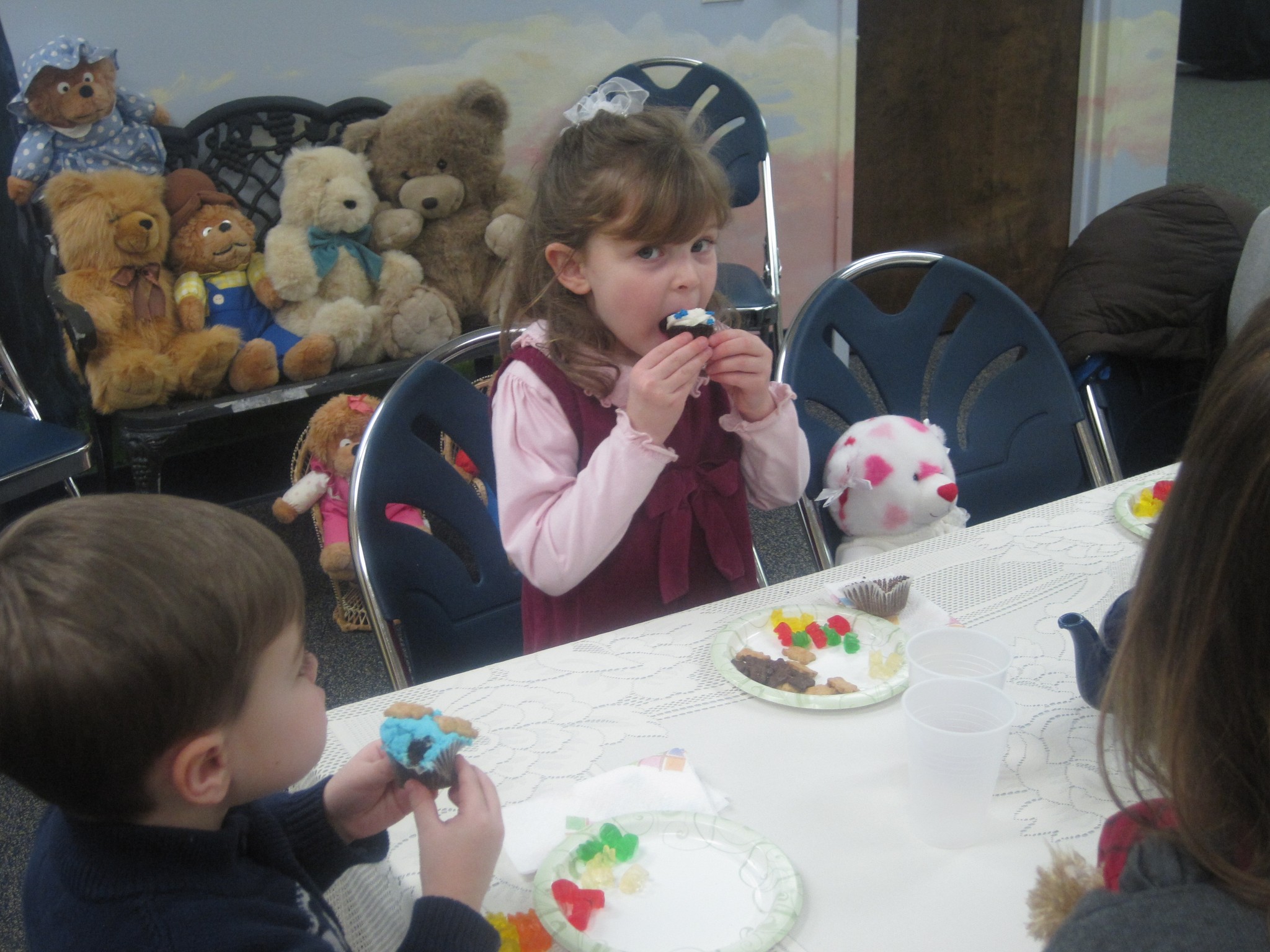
[1078,184,1246,473]
[0,338,94,510]
[348,317,778,691]
[588,52,786,379]
[784,247,1106,567]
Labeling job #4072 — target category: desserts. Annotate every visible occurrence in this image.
[668,305,715,377]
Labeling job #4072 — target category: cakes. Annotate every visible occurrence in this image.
[381,702,478,789]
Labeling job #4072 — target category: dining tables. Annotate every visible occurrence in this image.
[307,457,1189,952]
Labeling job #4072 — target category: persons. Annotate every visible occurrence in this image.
[1030,298,1270,950]
[486,77,813,656]
[1,496,506,952]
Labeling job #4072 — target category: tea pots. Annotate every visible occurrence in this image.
[1057,578,1159,714]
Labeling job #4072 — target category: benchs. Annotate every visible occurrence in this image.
[112,91,497,590]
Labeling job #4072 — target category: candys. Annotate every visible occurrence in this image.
[482,823,646,952]
[733,611,905,696]
[1133,478,1176,519]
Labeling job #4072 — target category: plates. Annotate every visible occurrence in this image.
[711,604,916,710]
[533,810,804,952]
[1113,477,1181,541]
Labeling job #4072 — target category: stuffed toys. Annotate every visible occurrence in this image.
[0,34,536,415]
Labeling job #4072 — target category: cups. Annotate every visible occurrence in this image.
[901,624,1019,850]
